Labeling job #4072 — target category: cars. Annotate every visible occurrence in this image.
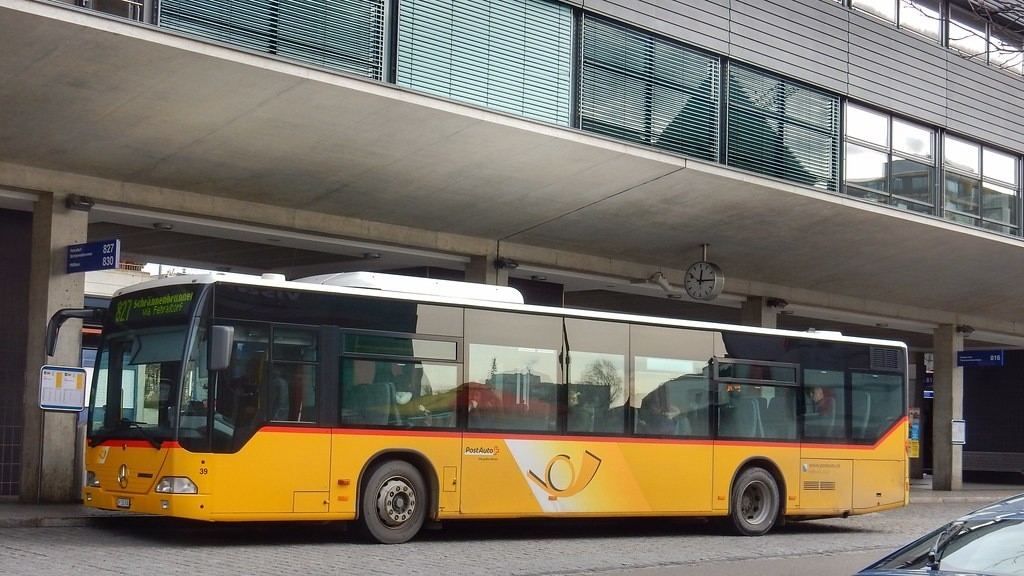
[852,492,1023,575]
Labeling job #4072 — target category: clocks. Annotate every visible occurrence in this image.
[683,261,726,302]
[923,353,934,371]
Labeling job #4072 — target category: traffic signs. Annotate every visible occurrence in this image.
[65,238,120,273]
[957,350,1003,366]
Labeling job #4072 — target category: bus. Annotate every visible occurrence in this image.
[43,269,910,548]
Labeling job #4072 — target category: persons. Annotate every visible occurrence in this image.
[806,386,833,432]
[187,346,686,441]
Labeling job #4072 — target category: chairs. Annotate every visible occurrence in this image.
[345,365,873,442]
